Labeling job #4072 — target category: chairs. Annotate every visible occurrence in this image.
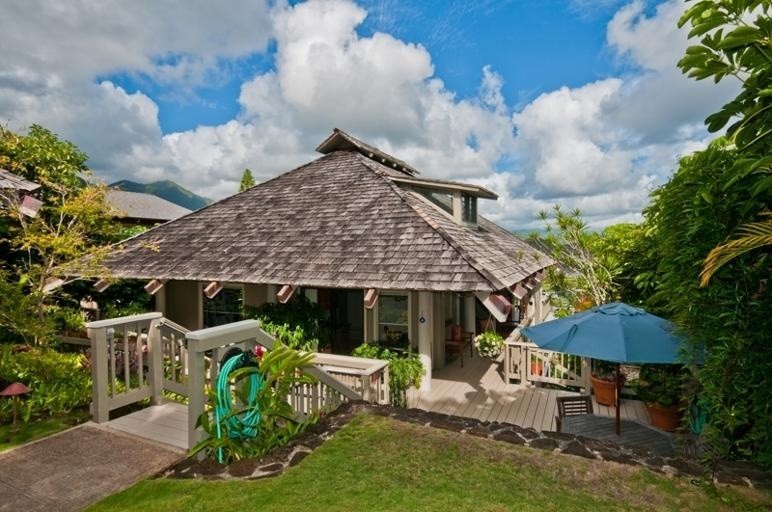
[555,395,594,432]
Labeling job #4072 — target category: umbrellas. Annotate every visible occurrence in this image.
[518,294,709,434]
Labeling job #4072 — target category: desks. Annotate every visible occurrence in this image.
[445,325,474,368]
[560,413,675,458]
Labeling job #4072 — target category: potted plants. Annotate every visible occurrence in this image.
[629,363,688,429]
[590,357,627,406]
